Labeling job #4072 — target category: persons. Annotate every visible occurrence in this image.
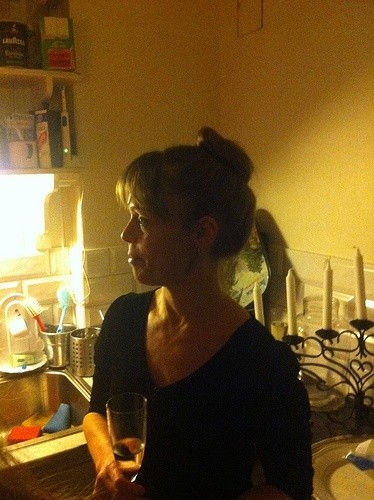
[82,127,315,500]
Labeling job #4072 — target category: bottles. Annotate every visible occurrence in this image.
[296,296,351,412]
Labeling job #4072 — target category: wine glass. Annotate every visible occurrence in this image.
[106,393,148,484]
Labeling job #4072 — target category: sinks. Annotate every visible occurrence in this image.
[0,365,93,455]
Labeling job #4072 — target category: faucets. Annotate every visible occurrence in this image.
[0,294,38,362]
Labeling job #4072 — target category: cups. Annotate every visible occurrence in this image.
[39,324,77,368]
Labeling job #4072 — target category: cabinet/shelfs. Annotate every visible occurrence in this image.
[0,66,83,234]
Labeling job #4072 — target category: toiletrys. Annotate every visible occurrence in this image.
[32,86,71,169]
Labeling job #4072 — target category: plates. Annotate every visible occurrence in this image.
[311,435,374,500]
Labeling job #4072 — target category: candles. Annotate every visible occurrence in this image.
[253,280,266,327]
[353,246,368,321]
[322,257,333,331]
[286,267,298,336]
